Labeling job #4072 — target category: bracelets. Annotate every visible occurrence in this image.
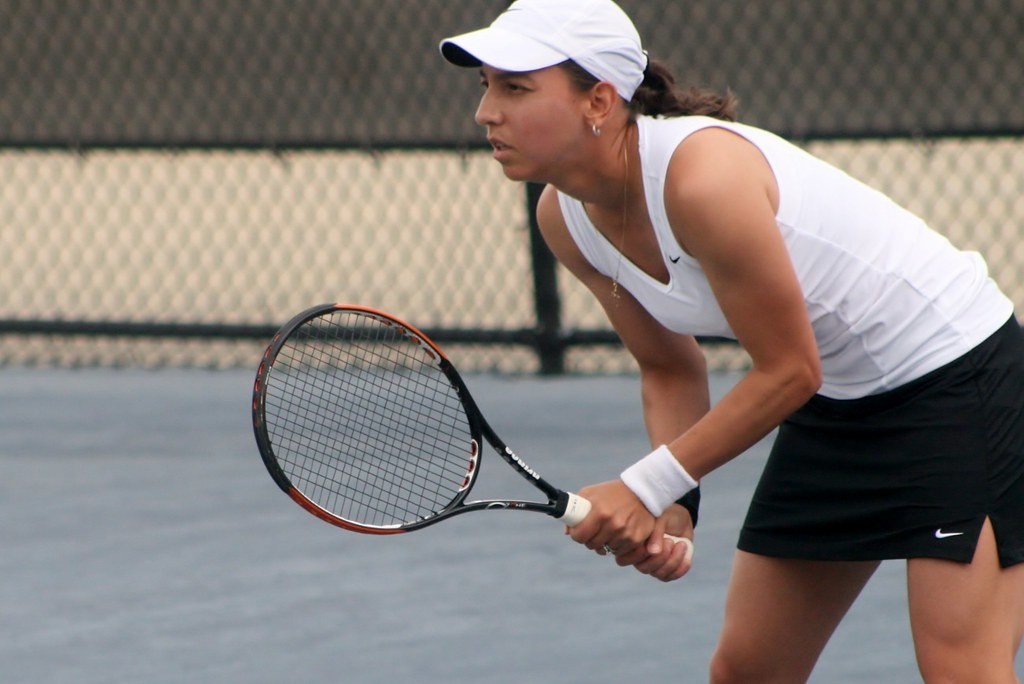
[676,488,701,530]
[619,446,700,517]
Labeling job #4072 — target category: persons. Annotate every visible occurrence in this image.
[439,0,1024,684]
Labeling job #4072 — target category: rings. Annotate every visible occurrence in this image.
[603,544,616,555]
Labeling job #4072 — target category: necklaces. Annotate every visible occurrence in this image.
[580,147,630,308]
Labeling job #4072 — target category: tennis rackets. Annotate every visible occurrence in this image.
[251,302,695,565]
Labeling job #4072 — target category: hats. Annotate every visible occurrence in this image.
[439,0,647,103]
